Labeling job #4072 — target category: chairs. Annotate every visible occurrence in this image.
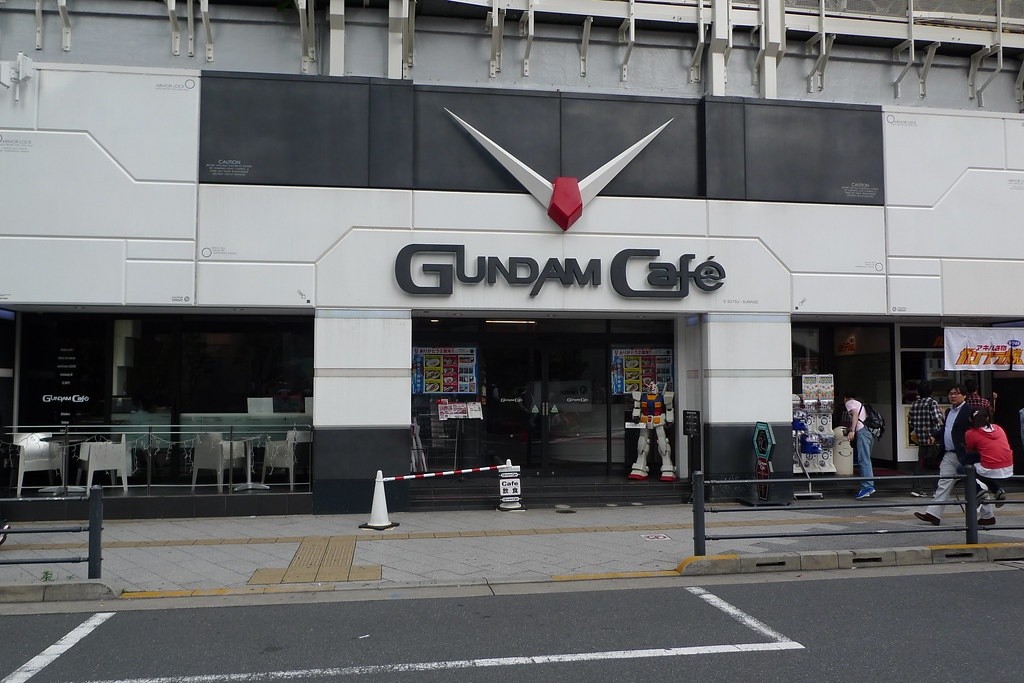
[76,433,128,497]
[261,431,313,492]
[13,432,65,498]
[191,432,245,494]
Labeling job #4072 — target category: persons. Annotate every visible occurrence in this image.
[956,408,1014,508]
[628,381,678,481]
[964,379,993,424]
[843,391,876,500]
[908,381,944,497]
[1019,408,1024,446]
[834,388,847,426]
[914,384,995,526]
[487,380,500,432]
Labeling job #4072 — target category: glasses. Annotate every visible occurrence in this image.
[948,391,962,395]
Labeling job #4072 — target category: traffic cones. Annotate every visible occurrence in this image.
[359,469,400,531]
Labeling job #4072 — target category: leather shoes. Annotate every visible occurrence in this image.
[914,512,940,525]
[977,516,996,525]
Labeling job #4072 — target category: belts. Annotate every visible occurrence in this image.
[945,450,954,452]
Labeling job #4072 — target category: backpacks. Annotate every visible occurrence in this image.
[850,400,886,442]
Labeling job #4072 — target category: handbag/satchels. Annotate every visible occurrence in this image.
[923,444,945,470]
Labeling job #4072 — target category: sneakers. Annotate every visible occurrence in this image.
[854,489,870,497]
[932,494,935,497]
[856,486,876,499]
[911,488,929,497]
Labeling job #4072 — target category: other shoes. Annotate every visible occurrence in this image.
[976,489,989,509]
[995,488,1006,508]
[0,522,10,545]
